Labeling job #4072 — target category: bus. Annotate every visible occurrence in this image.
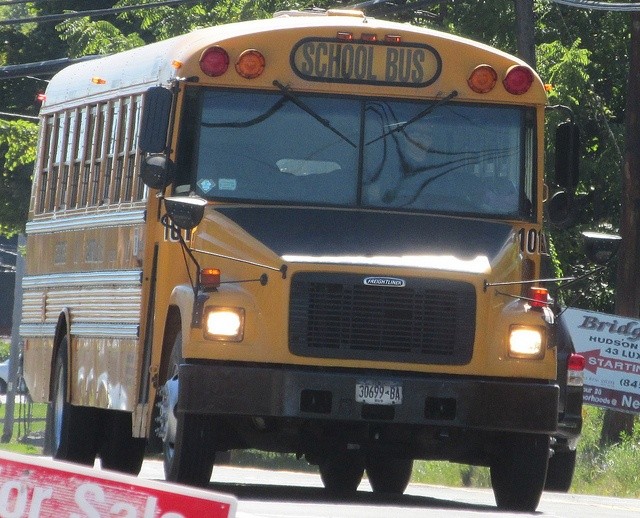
[19,5,561,512]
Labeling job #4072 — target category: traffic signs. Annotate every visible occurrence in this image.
[559,306,640,417]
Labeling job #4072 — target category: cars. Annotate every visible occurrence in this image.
[544,296,585,492]
[1,353,24,394]
